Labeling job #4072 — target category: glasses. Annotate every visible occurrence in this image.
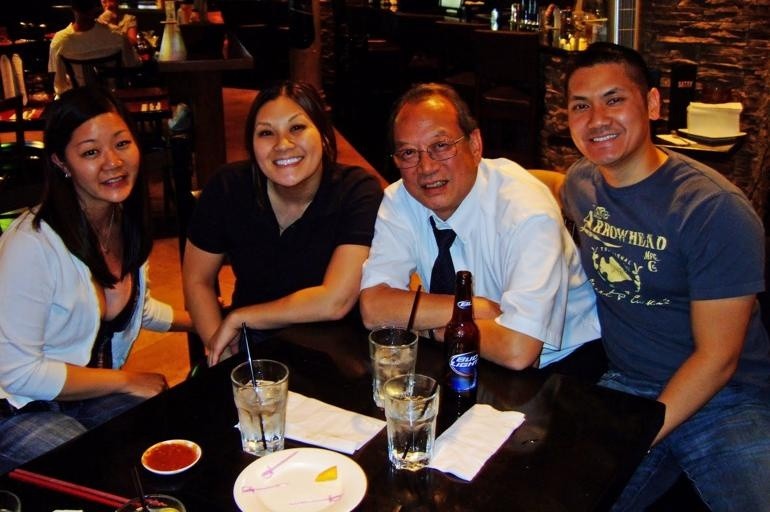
[390,134,467,168]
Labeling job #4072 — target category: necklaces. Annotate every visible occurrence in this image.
[79,204,122,255]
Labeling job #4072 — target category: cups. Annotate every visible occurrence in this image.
[115,495,187,511]
[0,490,21,512]
[230,359,289,457]
[369,325,419,408]
[383,374,440,471]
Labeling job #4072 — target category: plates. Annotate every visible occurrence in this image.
[141,438,202,474]
[232,447,368,512]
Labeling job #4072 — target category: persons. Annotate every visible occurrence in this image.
[175,1,199,25]
[221,1,241,61]
[557,41,770,512]
[47,0,136,95]
[359,83,604,376]
[95,1,138,47]
[0,83,195,474]
[181,81,384,370]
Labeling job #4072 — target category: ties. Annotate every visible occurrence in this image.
[430,215,457,295]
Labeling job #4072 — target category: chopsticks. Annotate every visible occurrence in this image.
[13,468,131,503]
[8,472,121,508]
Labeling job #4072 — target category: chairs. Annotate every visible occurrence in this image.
[54,46,128,90]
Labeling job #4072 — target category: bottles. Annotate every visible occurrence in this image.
[490,8,498,31]
[519,0,526,31]
[526,0,532,31]
[532,0,537,32]
[442,271,481,419]
